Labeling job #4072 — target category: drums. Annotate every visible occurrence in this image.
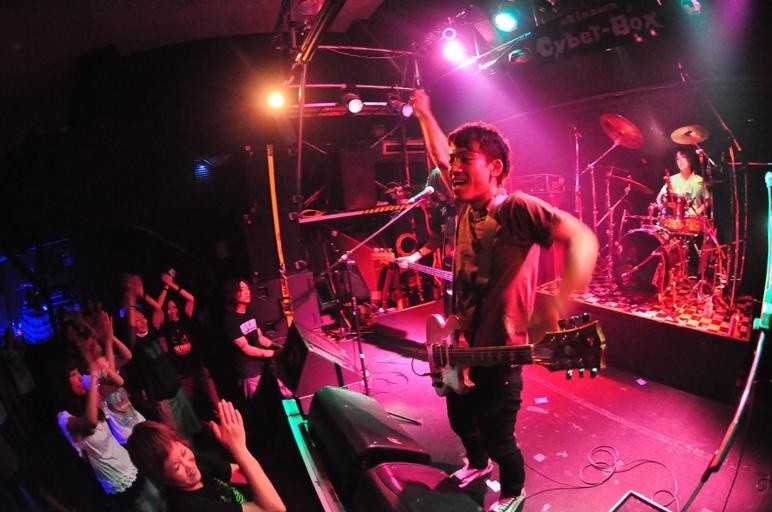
[685,216,706,235]
[658,194,686,232]
[612,226,685,306]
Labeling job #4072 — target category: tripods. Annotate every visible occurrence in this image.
[672,172,731,319]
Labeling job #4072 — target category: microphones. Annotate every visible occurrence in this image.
[408,185,434,202]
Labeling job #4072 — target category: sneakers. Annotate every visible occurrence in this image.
[489,488,526,512]
[448,459,493,489]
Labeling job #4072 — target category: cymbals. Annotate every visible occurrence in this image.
[602,115,643,150]
[597,165,630,176]
[698,180,721,184]
[671,125,710,146]
[613,175,654,196]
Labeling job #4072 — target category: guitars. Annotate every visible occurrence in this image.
[426,311,606,396]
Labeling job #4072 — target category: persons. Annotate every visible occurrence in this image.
[439,121,598,512]
[1,268,288,512]
[656,146,713,281]
[394,166,455,297]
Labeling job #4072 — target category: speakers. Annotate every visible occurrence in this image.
[279,318,361,395]
[306,385,433,499]
[362,461,449,510]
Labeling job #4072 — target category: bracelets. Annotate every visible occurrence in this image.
[416,250,425,258]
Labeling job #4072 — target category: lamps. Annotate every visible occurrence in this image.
[386,92,414,118]
[339,80,364,114]
